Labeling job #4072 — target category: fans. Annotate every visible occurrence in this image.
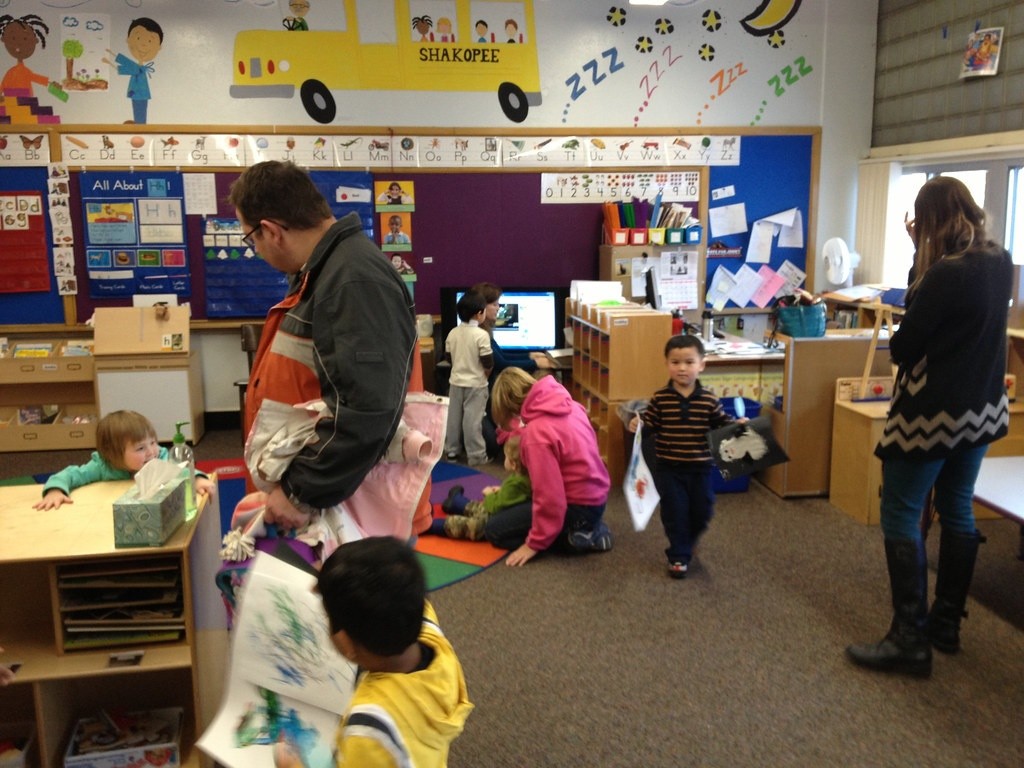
[822,237,861,285]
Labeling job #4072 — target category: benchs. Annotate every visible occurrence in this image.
[753,332,897,495]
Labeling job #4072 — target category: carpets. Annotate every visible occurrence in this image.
[196,457,512,593]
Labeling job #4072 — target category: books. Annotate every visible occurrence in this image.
[57,559,185,652]
[13,339,96,358]
[17,404,96,424]
[63,704,184,768]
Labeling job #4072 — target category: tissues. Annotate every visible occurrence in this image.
[112,458,189,547]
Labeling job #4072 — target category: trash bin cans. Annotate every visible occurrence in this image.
[624,399,655,474]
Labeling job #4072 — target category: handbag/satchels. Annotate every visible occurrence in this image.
[768,295,827,348]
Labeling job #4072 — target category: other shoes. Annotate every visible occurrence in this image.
[442,484,466,513]
[449,451,459,463]
[666,553,690,578]
[469,455,496,467]
[562,527,617,555]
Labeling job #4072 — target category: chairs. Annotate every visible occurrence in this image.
[234,324,264,448]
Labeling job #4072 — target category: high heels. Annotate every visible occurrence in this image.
[848,642,935,678]
[929,618,961,652]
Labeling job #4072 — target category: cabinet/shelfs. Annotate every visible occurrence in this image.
[0,337,95,455]
[565,296,674,488]
[95,350,206,447]
[0,471,229,768]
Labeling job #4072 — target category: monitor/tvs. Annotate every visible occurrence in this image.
[440,288,565,353]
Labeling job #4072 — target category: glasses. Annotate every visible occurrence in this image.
[242,219,290,252]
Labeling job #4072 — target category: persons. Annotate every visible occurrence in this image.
[843,176,1012,678]
[443,289,495,467]
[33,409,215,512]
[425,366,612,567]
[231,159,425,558]
[473,283,549,431]
[272,536,474,768]
[627,335,749,579]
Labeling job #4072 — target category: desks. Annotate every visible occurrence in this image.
[683,327,786,409]
[922,455,1024,542]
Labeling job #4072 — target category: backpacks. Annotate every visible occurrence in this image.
[216,524,323,632]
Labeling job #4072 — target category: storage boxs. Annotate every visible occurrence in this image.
[605,225,703,247]
[64,707,183,768]
[93,306,191,355]
